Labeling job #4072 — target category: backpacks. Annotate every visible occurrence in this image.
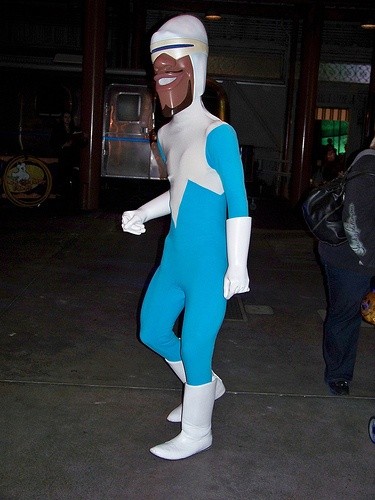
[301,166,375,247]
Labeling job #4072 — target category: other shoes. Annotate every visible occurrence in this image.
[325,378,351,394]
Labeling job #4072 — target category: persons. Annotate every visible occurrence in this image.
[48,111,89,217]
[326,138,337,158]
[122,13,254,462]
[319,136,375,395]
